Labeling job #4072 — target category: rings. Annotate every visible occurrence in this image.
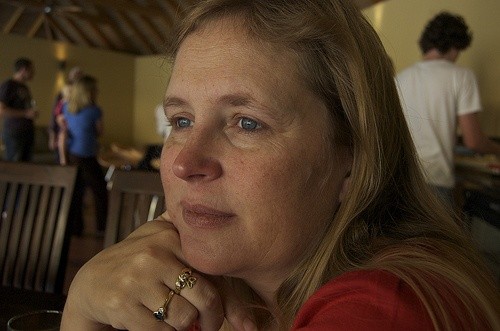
[152,269,196,321]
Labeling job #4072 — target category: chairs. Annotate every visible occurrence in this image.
[102,170,166,249]
[0,161,79,331]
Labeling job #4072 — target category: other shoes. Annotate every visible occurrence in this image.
[95,229,105,239]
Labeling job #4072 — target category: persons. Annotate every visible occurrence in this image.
[0,58,108,238]
[393,12,500,188]
[60,0,500,331]
